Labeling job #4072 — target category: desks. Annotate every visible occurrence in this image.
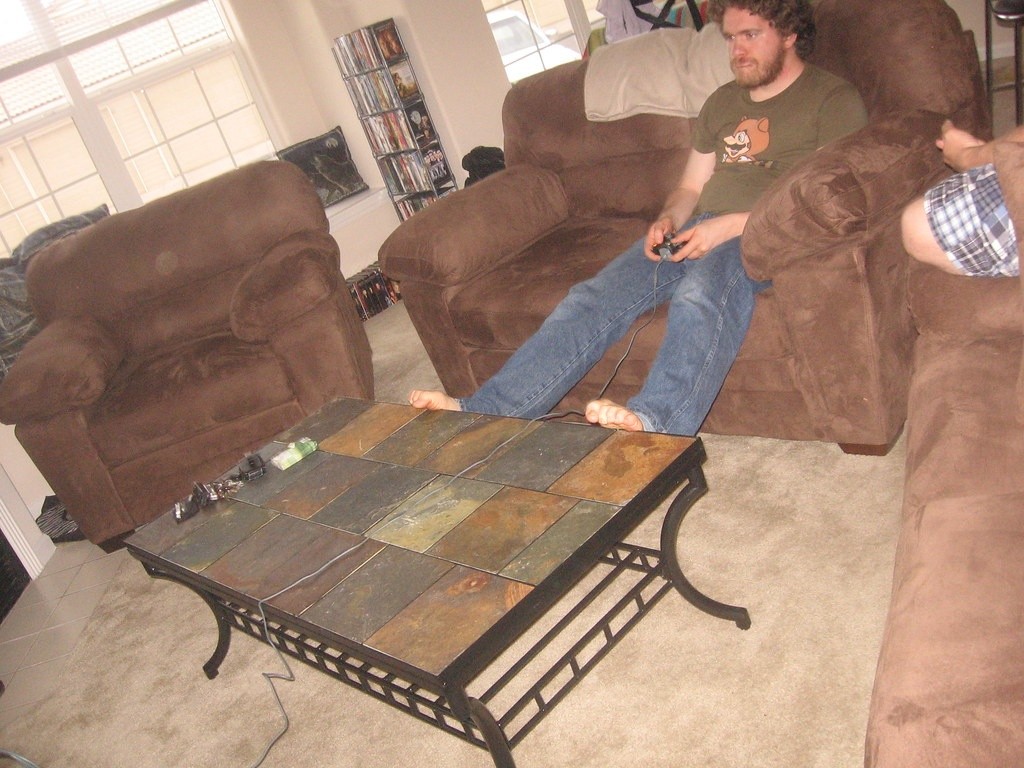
[421,573,432,592]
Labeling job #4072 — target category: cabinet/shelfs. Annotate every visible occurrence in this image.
[332,18,459,223]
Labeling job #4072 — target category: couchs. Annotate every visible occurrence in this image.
[0,161,375,554]
[864,124,1024,768]
[378,0,993,456]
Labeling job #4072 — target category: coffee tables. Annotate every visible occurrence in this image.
[123,396,751,768]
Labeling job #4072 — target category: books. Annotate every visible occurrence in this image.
[396,189,454,221]
[333,18,456,156]
[413,142,450,185]
[378,149,426,192]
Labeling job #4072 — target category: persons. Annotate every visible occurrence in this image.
[406,106,425,140]
[377,33,394,60]
[383,27,404,55]
[424,149,450,177]
[393,72,409,100]
[407,0,868,433]
[901,119,1024,277]
